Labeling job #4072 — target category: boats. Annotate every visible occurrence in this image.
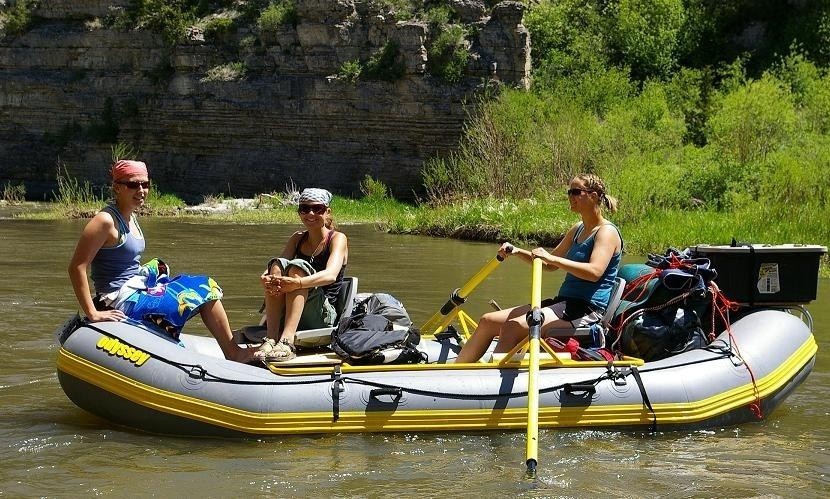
[52,299,820,440]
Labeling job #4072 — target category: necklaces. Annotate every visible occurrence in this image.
[307,231,327,264]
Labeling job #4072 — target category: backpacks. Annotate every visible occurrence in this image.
[327,313,428,366]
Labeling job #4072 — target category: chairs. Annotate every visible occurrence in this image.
[543,276,626,338]
[241,277,358,348]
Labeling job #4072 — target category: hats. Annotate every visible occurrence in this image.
[112,160,148,183]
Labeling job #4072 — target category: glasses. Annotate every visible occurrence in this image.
[299,204,327,214]
[568,189,591,195]
[118,181,148,190]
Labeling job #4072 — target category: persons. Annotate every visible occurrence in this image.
[69,160,260,363]
[453,174,623,364]
[252,188,348,361]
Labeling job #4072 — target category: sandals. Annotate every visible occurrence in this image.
[254,337,297,362]
